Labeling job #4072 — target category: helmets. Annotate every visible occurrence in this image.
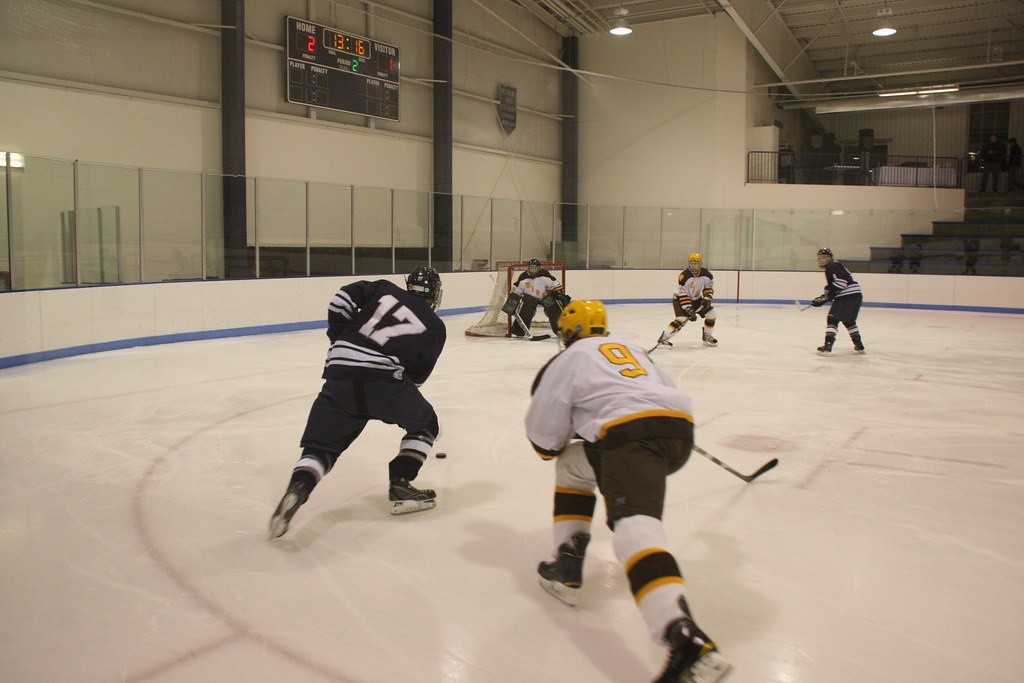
[816,247,834,269]
[527,259,541,277]
[687,253,704,277]
[403,265,443,312]
[555,299,610,346]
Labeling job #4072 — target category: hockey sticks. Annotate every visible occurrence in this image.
[690,441,779,483]
[514,310,551,341]
[794,299,826,311]
[647,304,705,354]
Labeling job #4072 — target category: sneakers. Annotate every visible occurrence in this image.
[816,336,836,356]
[852,335,865,354]
[648,596,734,683]
[701,327,718,347]
[656,331,673,348]
[387,474,438,515]
[535,532,592,606]
[266,479,312,539]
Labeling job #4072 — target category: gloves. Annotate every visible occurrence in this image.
[810,294,833,307]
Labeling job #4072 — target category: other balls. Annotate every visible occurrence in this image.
[436,452,447,459]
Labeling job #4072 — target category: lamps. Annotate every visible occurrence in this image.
[872,15,897,36]
[610,8,633,36]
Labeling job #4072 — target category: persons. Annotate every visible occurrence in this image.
[522,300,732,683]
[502,259,564,339]
[1007,137,1024,192]
[976,134,1006,197]
[268,265,447,542]
[656,252,718,350]
[811,247,865,357]
